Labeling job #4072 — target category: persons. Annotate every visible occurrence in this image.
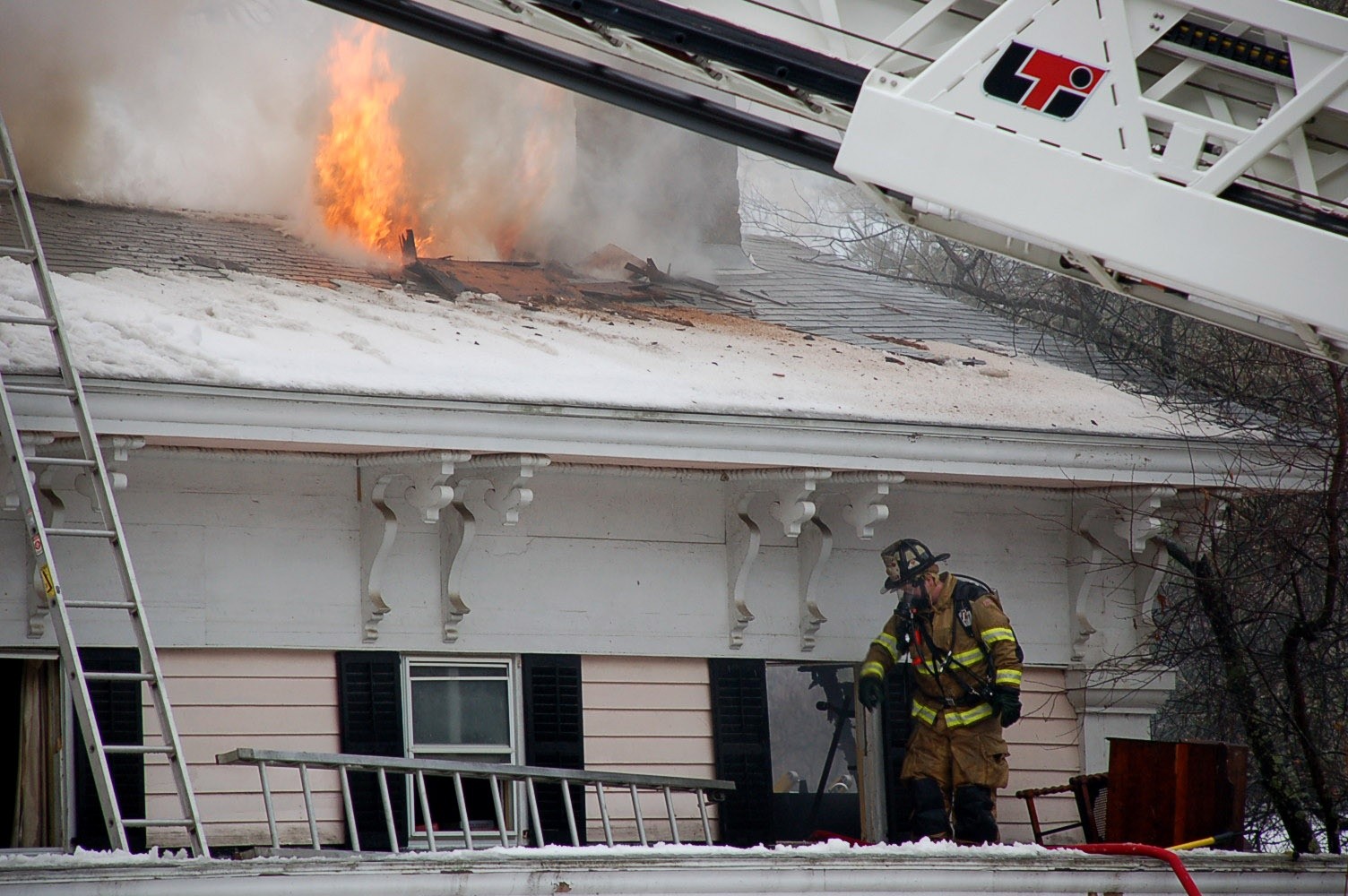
[858,538,1024,844]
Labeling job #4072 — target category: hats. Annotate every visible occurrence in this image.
[881,538,949,593]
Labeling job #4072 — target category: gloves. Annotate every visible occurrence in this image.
[858,672,881,711]
[990,687,1022,728]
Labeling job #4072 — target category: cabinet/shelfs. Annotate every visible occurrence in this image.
[1106,736,1250,852]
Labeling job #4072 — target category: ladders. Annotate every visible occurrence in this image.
[216,723,737,859]
[1,109,211,856]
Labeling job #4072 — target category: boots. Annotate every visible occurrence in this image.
[952,783,1000,846]
[887,777,949,842]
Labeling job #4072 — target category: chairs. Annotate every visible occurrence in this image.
[1016,772,1109,845]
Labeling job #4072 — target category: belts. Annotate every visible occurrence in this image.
[917,690,978,708]
